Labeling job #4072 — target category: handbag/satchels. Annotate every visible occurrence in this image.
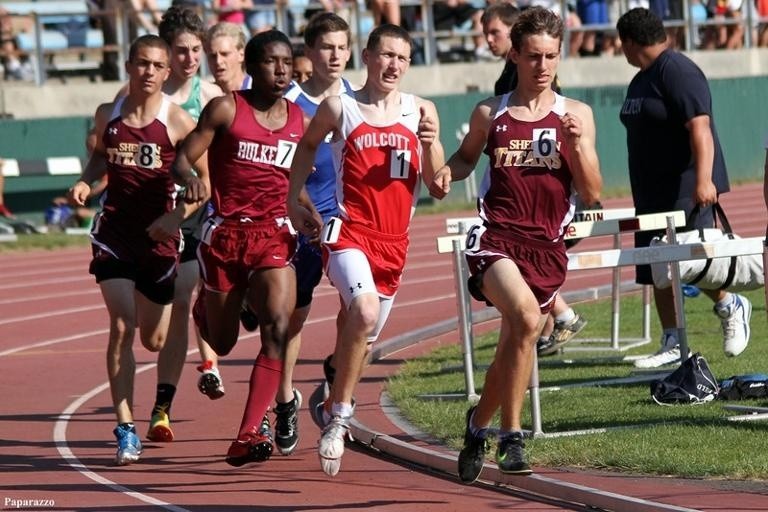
[652,194,767,294]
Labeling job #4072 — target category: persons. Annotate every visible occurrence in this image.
[615,7,752,369]
[0,1,768,84]
[196,21,254,400]
[113,5,227,442]
[171,30,323,466]
[269,12,363,456]
[53,132,108,219]
[290,42,312,84]
[431,8,603,486]
[66,33,213,467]
[286,25,445,479]
[481,3,588,356]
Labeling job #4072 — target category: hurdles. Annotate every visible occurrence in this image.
[488,235,768,441]
[414,209,690,407]
[445,208,661,362]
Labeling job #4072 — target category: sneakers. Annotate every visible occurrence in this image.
[145,404,176,445]
[195,366,225,401]
[632,333,693,369]
[110,419,143,465]
[493,430,534,475]
[456,404,490,485]
[539,309,588,357]
[314,354,357,478]
[713,290,753,359]
[222,388,303,469]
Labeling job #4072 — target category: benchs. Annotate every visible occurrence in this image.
[0,1,174,85]
